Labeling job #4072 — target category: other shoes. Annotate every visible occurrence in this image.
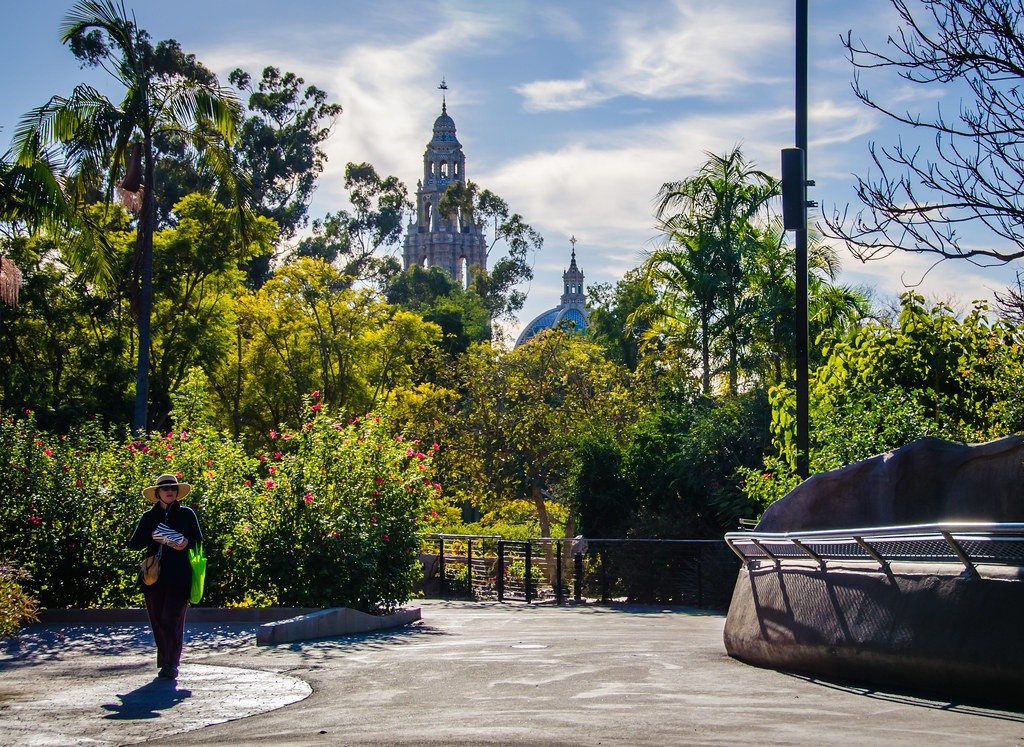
[158,666,179,679]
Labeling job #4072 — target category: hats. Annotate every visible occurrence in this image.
[142,474,192,502]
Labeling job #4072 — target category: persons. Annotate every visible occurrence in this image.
[129,473,202,677]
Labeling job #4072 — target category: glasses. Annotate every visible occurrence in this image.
[159,486,178,491]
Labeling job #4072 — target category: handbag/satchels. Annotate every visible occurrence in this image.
[188,542,207,604]
[140,555,160,585]
